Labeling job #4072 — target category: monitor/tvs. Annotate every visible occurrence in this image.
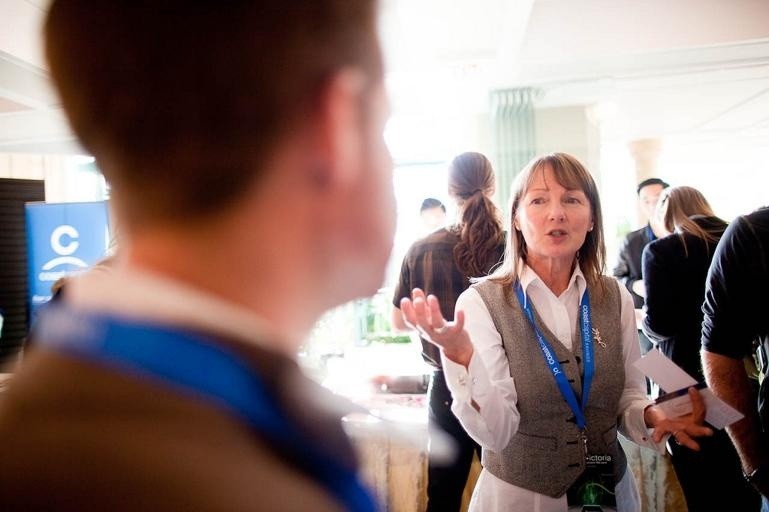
[24,198,108,352]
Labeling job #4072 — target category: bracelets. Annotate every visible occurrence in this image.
[741,461,769,484]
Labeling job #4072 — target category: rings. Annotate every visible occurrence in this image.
[672,430,681,437]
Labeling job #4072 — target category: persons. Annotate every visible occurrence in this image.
[697,206,769,512]
[419,198,446,231]
[388,153,510,510]
[604,176,675,400]
[637,186,765,512]
[397,150,715,511]
[0,1,407,511]
